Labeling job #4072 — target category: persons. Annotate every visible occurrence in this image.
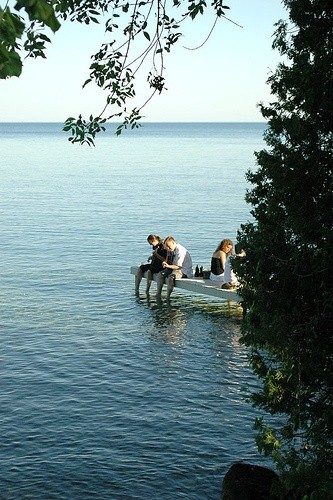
[133,234,167,295]
[224,243,246,285]
[153,235,193,302]
[209,238,234,282]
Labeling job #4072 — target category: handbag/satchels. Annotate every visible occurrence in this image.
[160,268,173,278]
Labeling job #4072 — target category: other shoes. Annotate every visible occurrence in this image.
[193,265,211,279]
